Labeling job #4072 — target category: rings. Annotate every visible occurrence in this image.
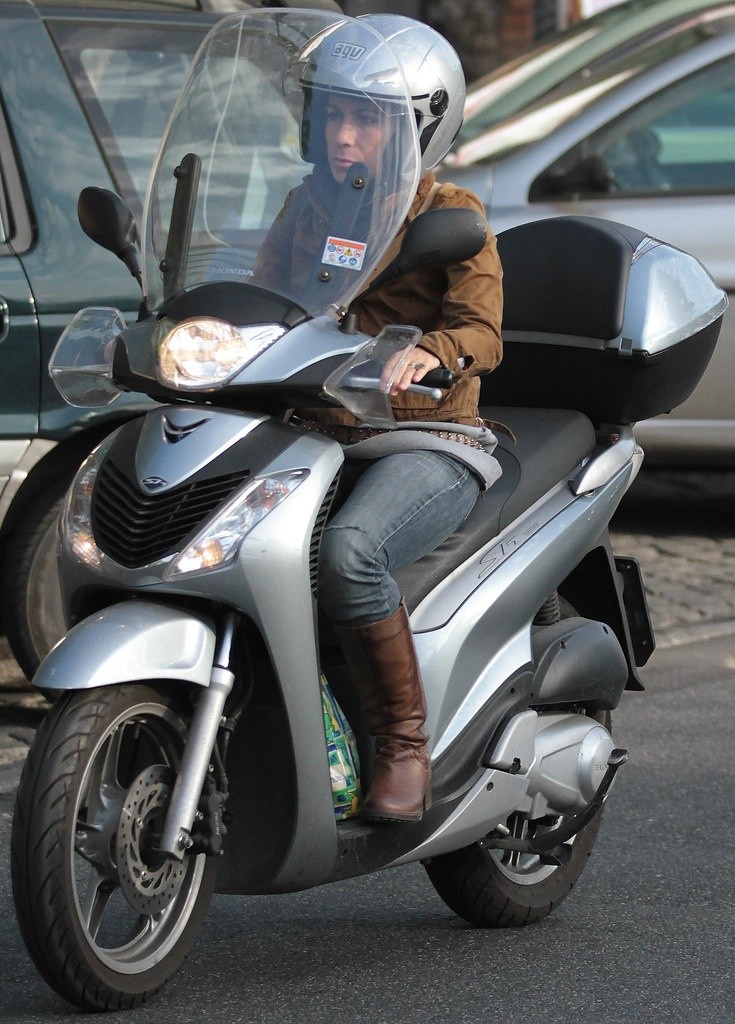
[408,363,416,367]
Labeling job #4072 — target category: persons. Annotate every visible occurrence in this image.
[243,14,502,822]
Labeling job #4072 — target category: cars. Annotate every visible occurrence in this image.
[431,1,735,454]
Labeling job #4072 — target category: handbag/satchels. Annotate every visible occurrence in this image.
[319,669,363,821]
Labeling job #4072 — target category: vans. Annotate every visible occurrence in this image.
[0,0,314,532]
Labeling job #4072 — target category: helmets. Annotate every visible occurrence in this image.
[295,13,466,184]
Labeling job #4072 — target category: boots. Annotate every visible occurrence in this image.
[332,596,432,823]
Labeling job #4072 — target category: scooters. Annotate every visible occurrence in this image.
[5,9,728,1010]
